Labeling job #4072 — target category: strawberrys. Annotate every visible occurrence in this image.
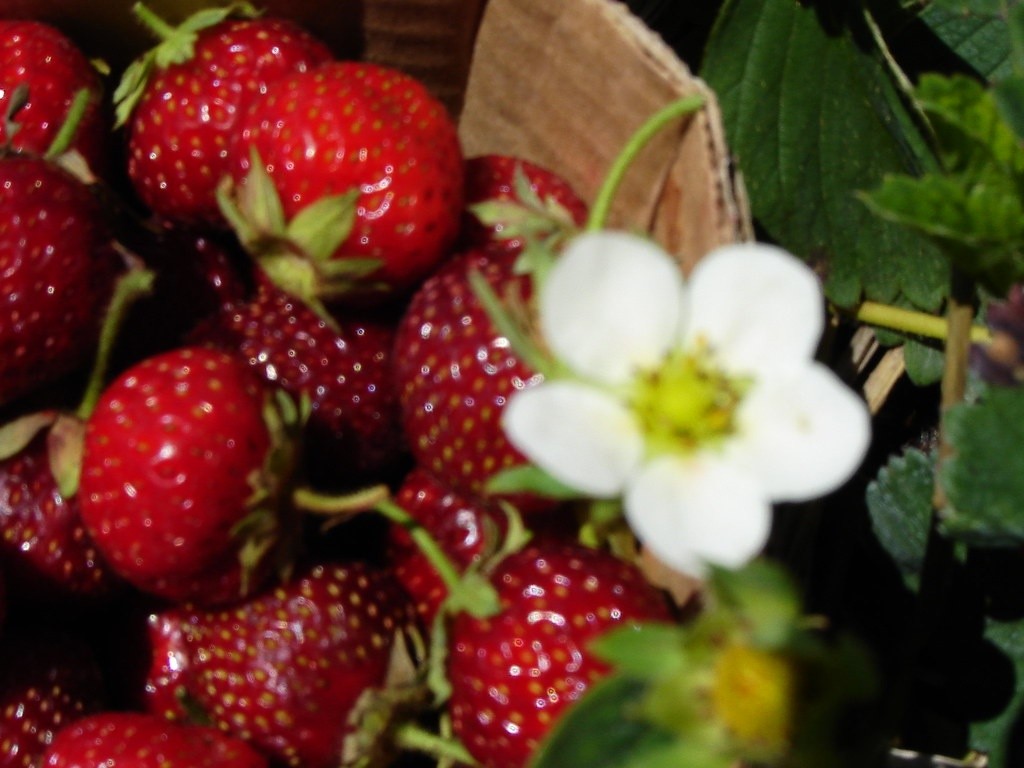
[0,0,681,768]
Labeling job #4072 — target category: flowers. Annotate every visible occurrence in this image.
[488,229,876,583]
